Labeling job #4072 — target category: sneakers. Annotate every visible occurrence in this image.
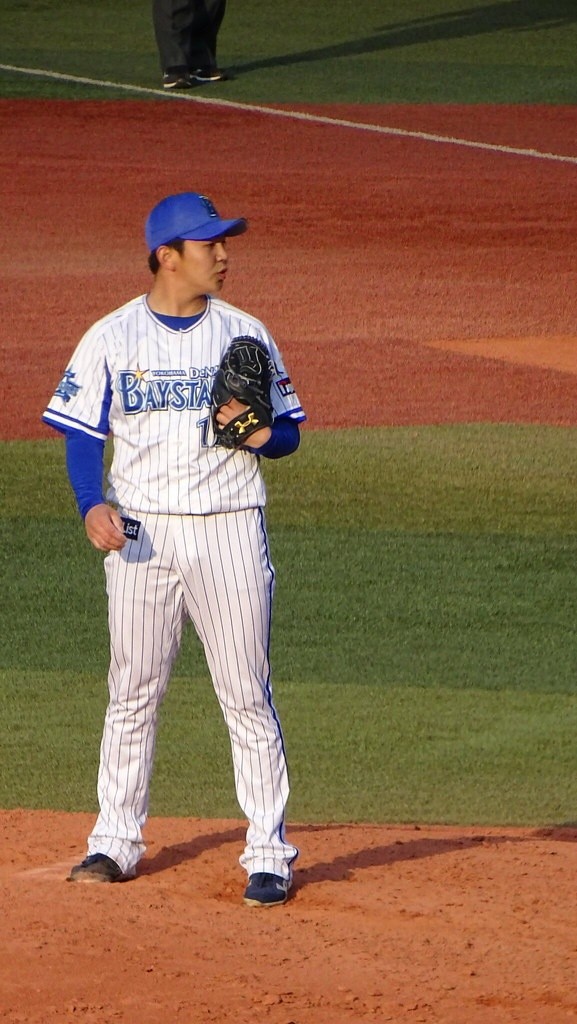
[188,66,223,82]
[70,852,134,885]
[161,68,191,89]
[243,872,293,906]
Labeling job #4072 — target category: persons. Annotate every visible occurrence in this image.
[153,0,227,88]
[40,190,306,906]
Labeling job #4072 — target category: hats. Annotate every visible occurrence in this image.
[145,193,250,250]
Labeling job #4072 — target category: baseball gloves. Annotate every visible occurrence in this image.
[208,334,275,450]
